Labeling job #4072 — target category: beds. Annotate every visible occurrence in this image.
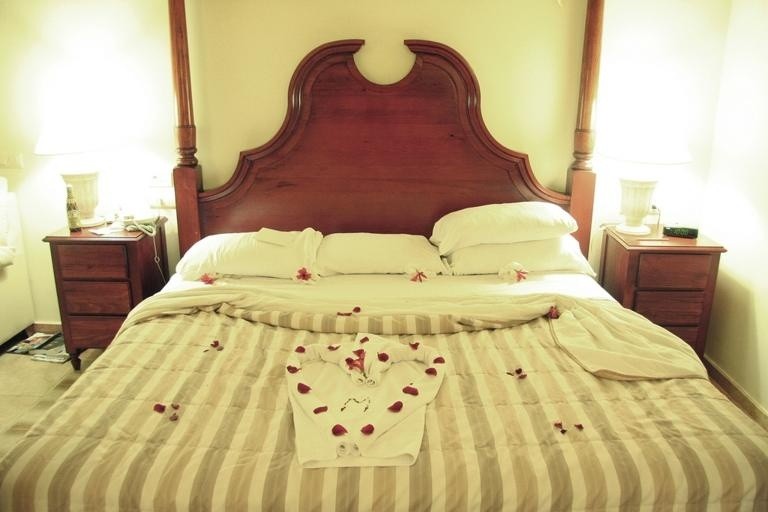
[0,0,767,512]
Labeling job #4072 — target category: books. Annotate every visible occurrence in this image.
[6,329,72,363]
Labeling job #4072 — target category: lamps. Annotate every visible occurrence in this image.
[615,162,658,237]
[61,140,106,228]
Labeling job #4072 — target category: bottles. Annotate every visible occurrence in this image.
[66,184,82,232]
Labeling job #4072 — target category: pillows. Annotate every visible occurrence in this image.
[317,233,447,278]
[442,234,597,279]
[429,201,579,258]
[175,230,324,282]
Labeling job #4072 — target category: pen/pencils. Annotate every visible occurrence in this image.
[636,237,669,240]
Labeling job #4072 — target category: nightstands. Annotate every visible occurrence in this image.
[42,217,169,370]
[599,223,728,360]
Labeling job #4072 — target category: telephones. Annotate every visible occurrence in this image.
[123,211,160,226]
